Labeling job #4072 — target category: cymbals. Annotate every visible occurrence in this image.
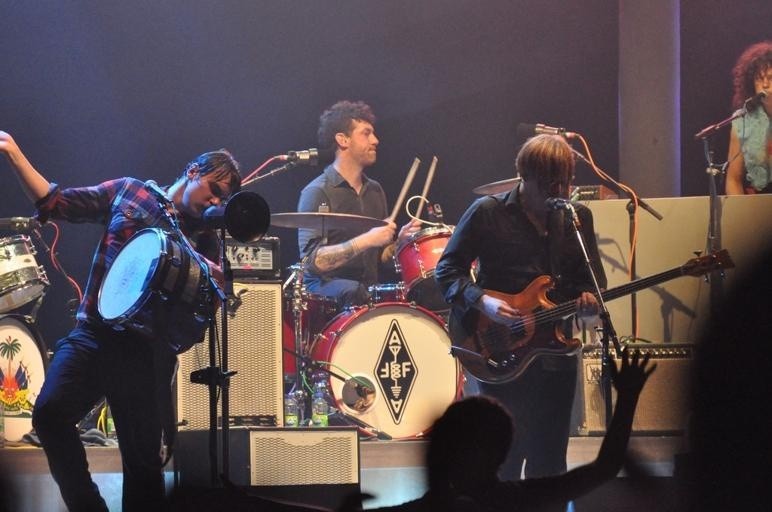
[269,201,390,232]
[471,176,525,196]
[0,213,47,236]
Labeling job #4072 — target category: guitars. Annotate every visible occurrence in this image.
[447,248,737,386]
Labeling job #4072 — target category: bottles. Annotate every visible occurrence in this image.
[313,392,327,426]
[105,405,117,440]
[285,393,298,426]
[296,390,306,426]
[310,393,317,427]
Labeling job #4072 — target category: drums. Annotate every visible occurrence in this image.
[301,300,467,442]
[394,225,480,313]
[1,311,54,443]
[96,226,224,358]
[1,234,52,316]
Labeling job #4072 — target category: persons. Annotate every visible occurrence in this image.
[726,41,771,195]
[361,344,659,512]
[0,129,241,510]
[297,99,422,314]
[435,133,607,510]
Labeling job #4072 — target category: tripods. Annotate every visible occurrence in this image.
[159,204,228,484]
[565,212,621,430]
[597,249,698,341]
[283,236,393,441]
[574,150,663,341]
[695,109,748,312]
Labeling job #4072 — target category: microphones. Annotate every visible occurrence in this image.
[515,123,567,138]
[145,179,173,205]
[281,147,319,167]
[544,198,574,210]
[742,90,767,116]
